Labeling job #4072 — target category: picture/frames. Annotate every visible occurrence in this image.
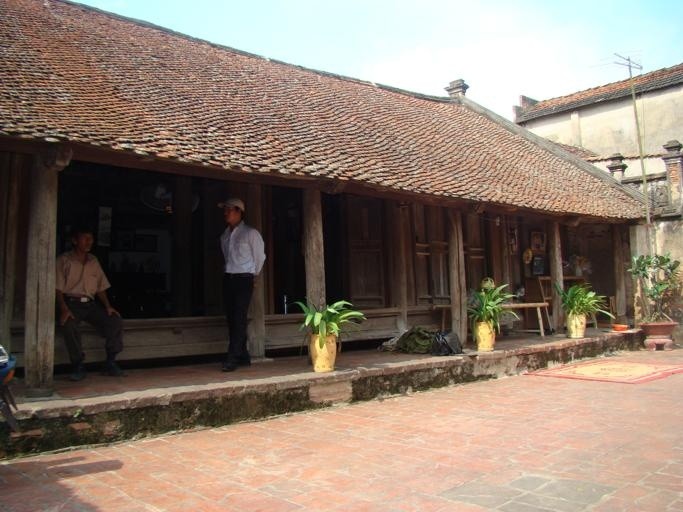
[529,230,548,276]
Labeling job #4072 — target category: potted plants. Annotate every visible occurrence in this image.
[626,252,681,351]
[286,295,368,373]
[467,280,521,353]
[554,279,616,338]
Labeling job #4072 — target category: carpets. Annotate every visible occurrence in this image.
[523,358,683,384]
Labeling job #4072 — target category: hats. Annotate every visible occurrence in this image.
[217,198,245,212]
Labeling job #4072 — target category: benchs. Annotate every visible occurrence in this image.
[432,303,550,342]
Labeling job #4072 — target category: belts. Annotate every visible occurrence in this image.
[224,273,253,278]
[65,297,91,304]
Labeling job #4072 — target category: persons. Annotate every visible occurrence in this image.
[218,198,266,372]
[56,224,128,381]
